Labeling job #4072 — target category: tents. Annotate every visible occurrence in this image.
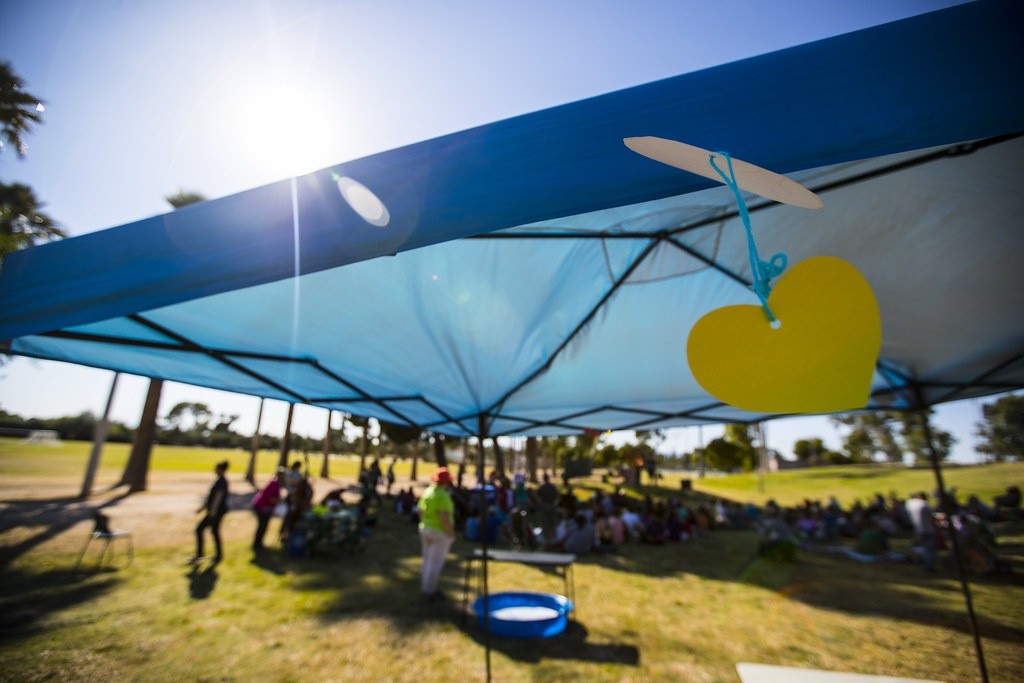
[0,0,1024,683]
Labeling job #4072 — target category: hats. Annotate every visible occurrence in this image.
[433,467,453,483]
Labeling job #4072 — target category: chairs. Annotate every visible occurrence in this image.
[74,508,132,570]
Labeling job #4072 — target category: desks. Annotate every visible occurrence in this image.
[462,548,577,625]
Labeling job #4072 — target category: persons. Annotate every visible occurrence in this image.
[188,461,229,563]
[251,450,1024,573]
[418,466,455,601]
